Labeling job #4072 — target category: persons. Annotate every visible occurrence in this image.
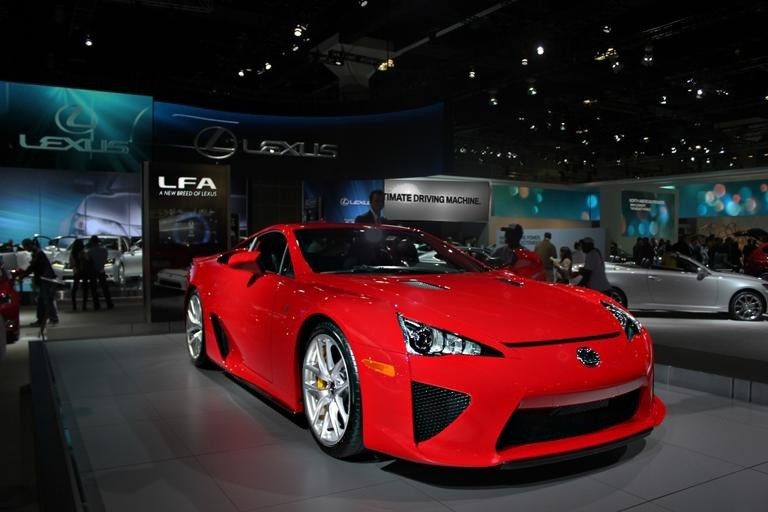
[8,235,114,328]
[353,190,390,237]
[632,234,757,269]
[449,224,613,298]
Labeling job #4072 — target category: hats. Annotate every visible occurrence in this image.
[501,223,523,236]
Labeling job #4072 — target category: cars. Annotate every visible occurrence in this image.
[569,242,768,321]
[416,240,500,267]
[153,268,187,291]
[0,234,143,341]
[184,224,667,470]
[69,173,142,237]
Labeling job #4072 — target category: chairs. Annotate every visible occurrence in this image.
[316,238,416,268]
